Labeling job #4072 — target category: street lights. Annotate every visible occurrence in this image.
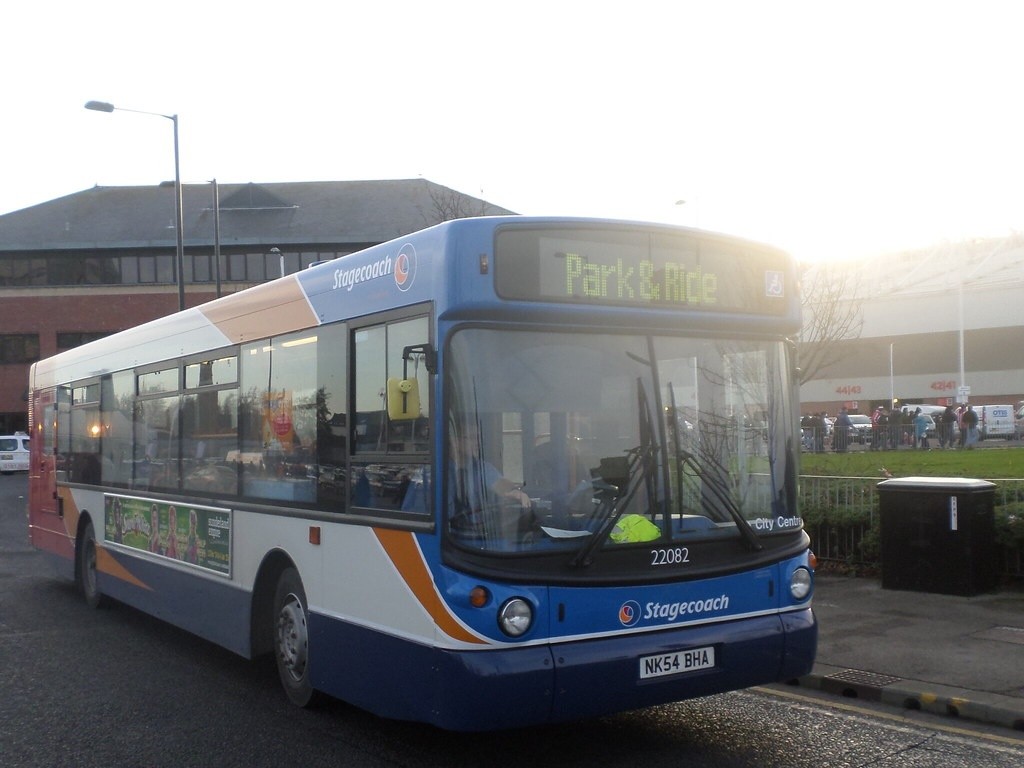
[270,247,286,278]
[83,99,185,314]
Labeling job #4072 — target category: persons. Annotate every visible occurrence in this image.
[352,408,602,527]
[112,498,201,565]
[801,404,979,453]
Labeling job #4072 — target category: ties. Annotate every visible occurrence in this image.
[454,469,467,508]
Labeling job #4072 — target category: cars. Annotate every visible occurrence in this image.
[183,464,238,495]
[298,464,430,502]
[800,414,873,442]
[1014,406,1024,439]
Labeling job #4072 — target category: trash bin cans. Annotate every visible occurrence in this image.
[874,477,997,599]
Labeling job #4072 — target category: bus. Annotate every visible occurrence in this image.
[28,214,819,733]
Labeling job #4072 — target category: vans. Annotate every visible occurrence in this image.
[0,432,32,472]
[953,405,1014,440]
[900,404,947,436]
